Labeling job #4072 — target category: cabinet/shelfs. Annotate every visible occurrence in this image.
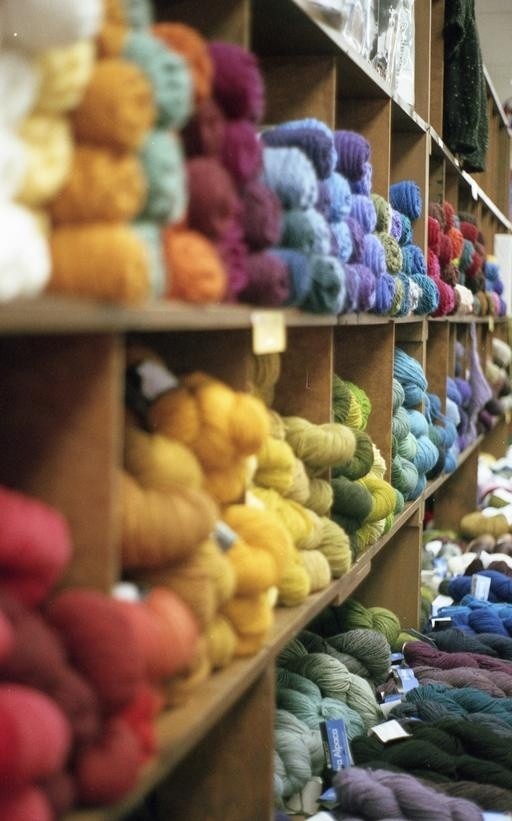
[0,0,510,820]
